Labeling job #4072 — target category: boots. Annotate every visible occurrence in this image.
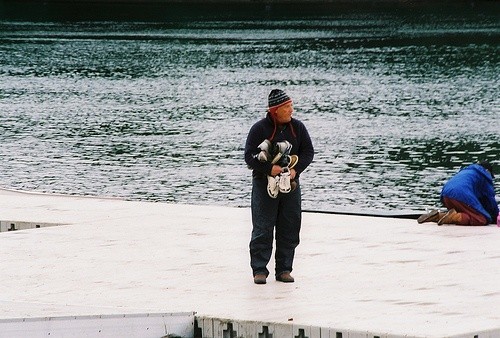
[438,209,462,226]
[417,209,446,224]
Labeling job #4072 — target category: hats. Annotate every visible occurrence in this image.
[268,89,292,118]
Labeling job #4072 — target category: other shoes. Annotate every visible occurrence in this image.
[291,181,299,193]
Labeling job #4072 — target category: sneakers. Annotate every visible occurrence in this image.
[254,274,266,284]
[255,139,298,170]
[279,167,291,193]
[276,271,294,282]
[267,174,279,198]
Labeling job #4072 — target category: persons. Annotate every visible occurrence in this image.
[244,88,315,283]
[417,163,499,226]
